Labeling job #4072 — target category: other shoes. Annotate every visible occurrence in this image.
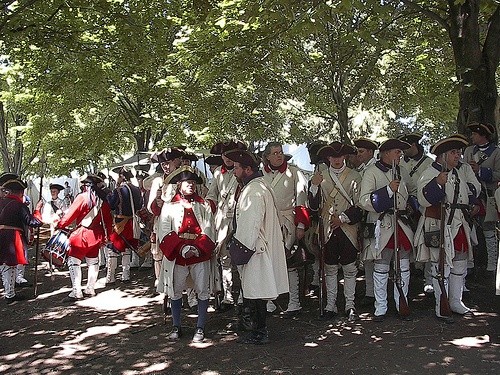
[224,317,251,329]
[118,274,131,284]
[15,279,28,286]
[237,327,270,344]
[130,250,140,268]
[169,325,181,339]
[105,278,121,287]
[5,292,28,303]
[361,294,375,306]
[373,309,388,322]
[192,326,205,343]
[393,308,413,322]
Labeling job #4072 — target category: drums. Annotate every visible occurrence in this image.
[40,228,74,270]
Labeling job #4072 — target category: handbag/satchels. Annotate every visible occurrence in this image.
[132,213,142,239]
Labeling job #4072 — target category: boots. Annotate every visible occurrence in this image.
[432,264,455,323]
[67,257,83,299]
[422,262,434,294]
[140,251,153,268]
[279,268,303,314]
[484,229,497,271]
[320,263,340,320]
[449,259,470,315]
[342,262,358,316]
[83,256,99,296]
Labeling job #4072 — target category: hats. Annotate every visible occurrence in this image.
[306,121,495,163]
[80,140,262,186]
[50,183,65,192]
[0,172,28,193]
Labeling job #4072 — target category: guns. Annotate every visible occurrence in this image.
[316,162,329,319]
[385,158,413,321]
[430,152,454,317]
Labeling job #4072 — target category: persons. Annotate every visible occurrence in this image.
[143,138,263,317]
[417,135,482,320]
[41,182,66,272]
[100,165,143,287]
[307,141,366,322]
[159,165,217,343]
[0,171,43,305]
[359,138,420,322]
[54,175,115,302]
[257,121,500,319]
[63,181,73,198]
[74,164,153,268]
[218,149,290,345]
